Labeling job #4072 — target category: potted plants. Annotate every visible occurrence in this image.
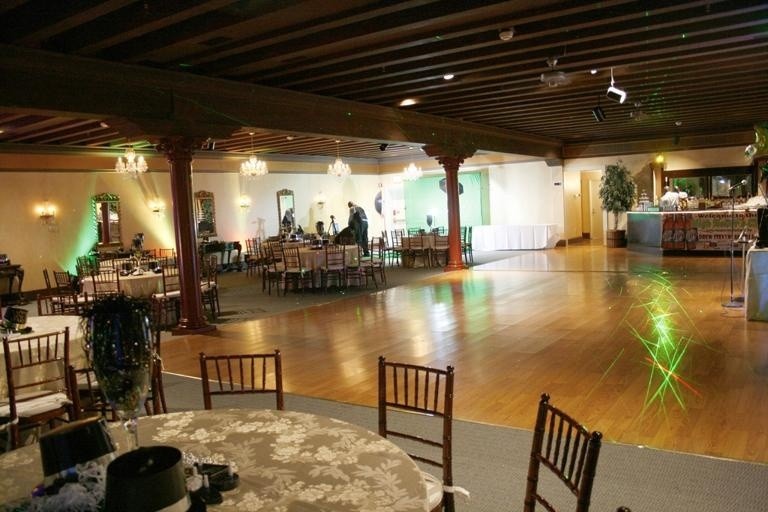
[599,161,638,248]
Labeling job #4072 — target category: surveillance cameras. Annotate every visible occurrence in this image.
[380,144,388,151]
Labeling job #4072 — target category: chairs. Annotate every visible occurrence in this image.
[152,264,180,326]
[89,269,121,301]
[394,228,406,246]
[252,237,261,255]
[78,256,95,275]
[347,239,378,286]
[379,231,394,265]
[460,226,473,264]
[1,326,80,451]
[199,255,220,319]
[377,354,454,512]
[312,239,329,246]
[244,239,259,277]
[148,256,167,273]
[267,241,288,296]
[159,248,174,259]
[259,242,282,293]
[138,297,169,413]
[459,226,467,263]
[53,270,95,315]
[433,227,452,269]
[390,230,408,266]
[524,391,602,512]
[36,268,60,315]
[319,242,346,292]
[363,237,387,283]
[65,352,168,423]
[95,258,114,272]
[113,258,138,271]
[199,351,284,410]
[280,245,316,296]
[141,248,157,260]
[408,227,429,269]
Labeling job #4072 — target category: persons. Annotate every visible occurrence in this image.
[282,209,293,232]
[673,187,688,202]
[312,221,328,240]
[327,215,341,235]
[347,201,369,257]
[659,186,680,210]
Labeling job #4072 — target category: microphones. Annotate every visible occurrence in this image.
[728,179,748,192]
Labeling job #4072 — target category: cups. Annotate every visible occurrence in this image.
[90,309,154,453]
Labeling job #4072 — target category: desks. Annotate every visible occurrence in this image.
[99,255,174,269]
[0,264,29,306]
[0,409,429,512]
[83,268,181,298]
[0,312,98,394]
[198,240,243,271]
[398,234,461,263]
[284,245,366,270]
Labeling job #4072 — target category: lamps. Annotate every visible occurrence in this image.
[541,59,566,88]
[239,195,251,207]
[403,154,423,181]
[328,141,352,178]
[316,192,328,207]
[36,199,56,226]
[149,199,164,212]
[239,129,268,179]
[115,139,147,175]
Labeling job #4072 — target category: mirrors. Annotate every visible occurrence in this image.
[277,188,295,233]
[93,193,123,251]
[194,191,218,237]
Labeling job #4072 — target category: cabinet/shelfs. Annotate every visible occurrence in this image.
[625,210,757,250]
